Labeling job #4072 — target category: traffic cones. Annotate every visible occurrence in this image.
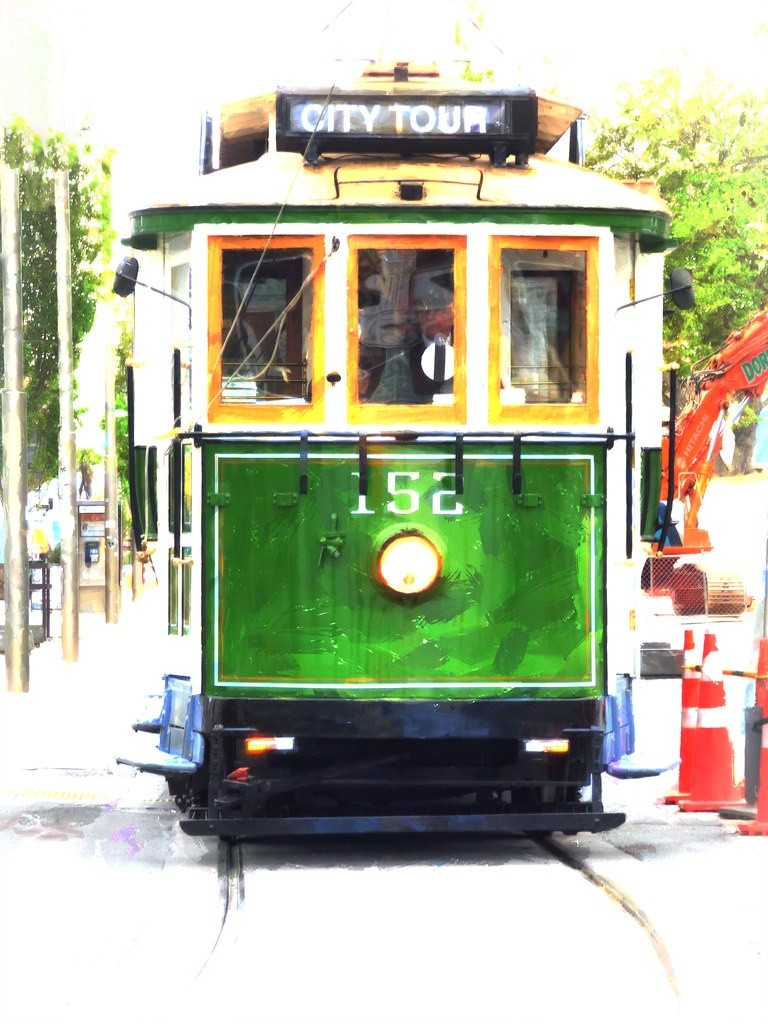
[663,630,702,803]
[737,639,768,837]
[678,633,746,813]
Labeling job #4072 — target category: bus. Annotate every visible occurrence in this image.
[105,62,699,841]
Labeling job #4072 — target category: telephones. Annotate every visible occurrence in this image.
[85,542,99,568]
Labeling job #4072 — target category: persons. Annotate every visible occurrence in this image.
[358,284,453,406]
